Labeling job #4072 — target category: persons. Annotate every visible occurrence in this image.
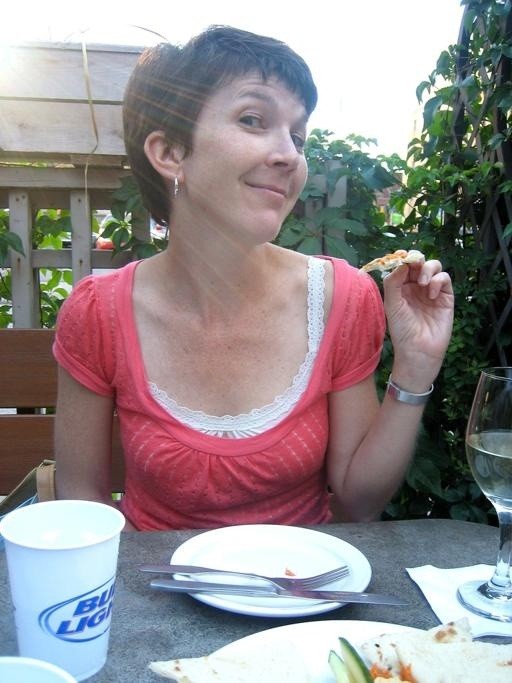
[42,20,456,537]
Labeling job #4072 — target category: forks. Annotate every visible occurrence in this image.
[139,561,349,592]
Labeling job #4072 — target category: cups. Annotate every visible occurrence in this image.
[0,499,126,681]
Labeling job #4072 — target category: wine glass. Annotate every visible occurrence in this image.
[458,365,512,625]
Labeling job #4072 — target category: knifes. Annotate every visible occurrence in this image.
[149,576,414,609]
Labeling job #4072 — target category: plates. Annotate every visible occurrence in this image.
[171,523,371,617]
[181,618,506,683]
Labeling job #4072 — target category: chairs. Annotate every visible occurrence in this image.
[0,326,127,510]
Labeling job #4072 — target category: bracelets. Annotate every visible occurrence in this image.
[383,372,434,406]
